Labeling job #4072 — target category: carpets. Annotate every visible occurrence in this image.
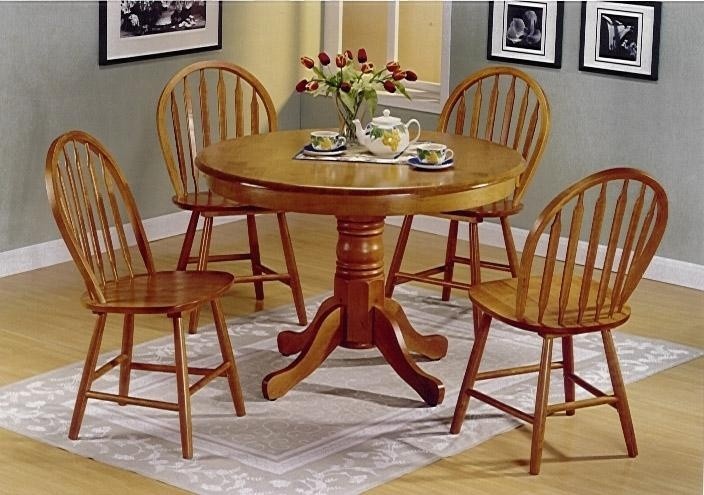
[0,283,704,495]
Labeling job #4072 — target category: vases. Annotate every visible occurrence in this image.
[339,118,362,147]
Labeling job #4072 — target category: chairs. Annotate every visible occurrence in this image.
[449,167,669,476]
[385,67,549,302]
[44,132,245,461]
[157,61,308,334]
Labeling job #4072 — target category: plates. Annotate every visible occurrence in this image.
[301,144,347,156]
[406,158,455,172]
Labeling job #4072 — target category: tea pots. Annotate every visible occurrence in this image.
[352,108,420,159]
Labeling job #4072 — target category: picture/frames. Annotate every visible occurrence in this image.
[487,1,564,69]
[97,0,223,66]
[579,0,662,81]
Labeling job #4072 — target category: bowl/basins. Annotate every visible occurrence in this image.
[416,143,455,164]
[309,130,346,151]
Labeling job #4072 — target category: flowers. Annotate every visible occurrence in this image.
[295,48,417,115]
